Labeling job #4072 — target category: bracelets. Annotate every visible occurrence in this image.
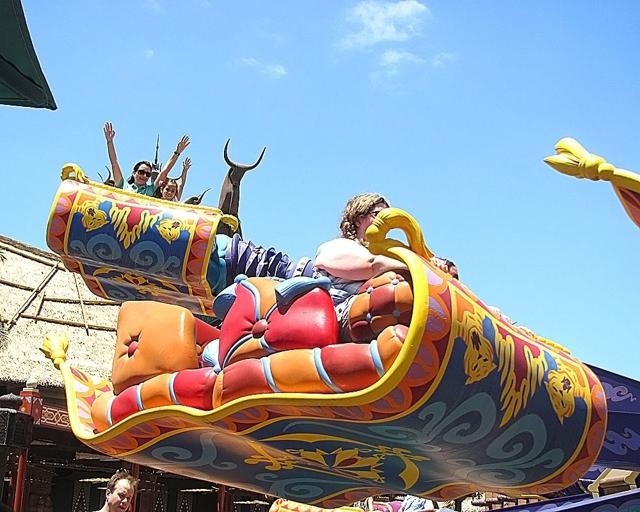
[174,151,180,156]
[107,140,112,144]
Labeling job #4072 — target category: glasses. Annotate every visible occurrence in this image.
[138,170,151,177]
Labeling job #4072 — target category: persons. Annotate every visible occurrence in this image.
[157,157,192,202]
[397,495,434,512]
[98,472,135,512]
[353,496,374,511]
[103,121,190,196]
[310,193,450,342]
